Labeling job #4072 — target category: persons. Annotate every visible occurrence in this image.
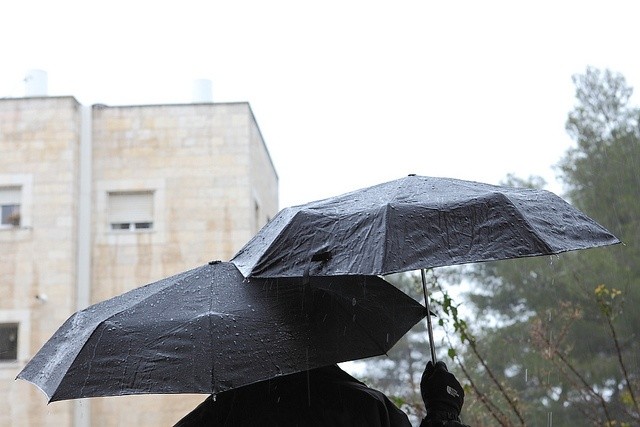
[173,361,465,426]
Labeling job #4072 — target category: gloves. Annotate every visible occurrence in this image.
[420,361,465,417]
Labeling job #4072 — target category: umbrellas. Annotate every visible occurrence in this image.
[14,261,428,405]
[234,175,618,384]
